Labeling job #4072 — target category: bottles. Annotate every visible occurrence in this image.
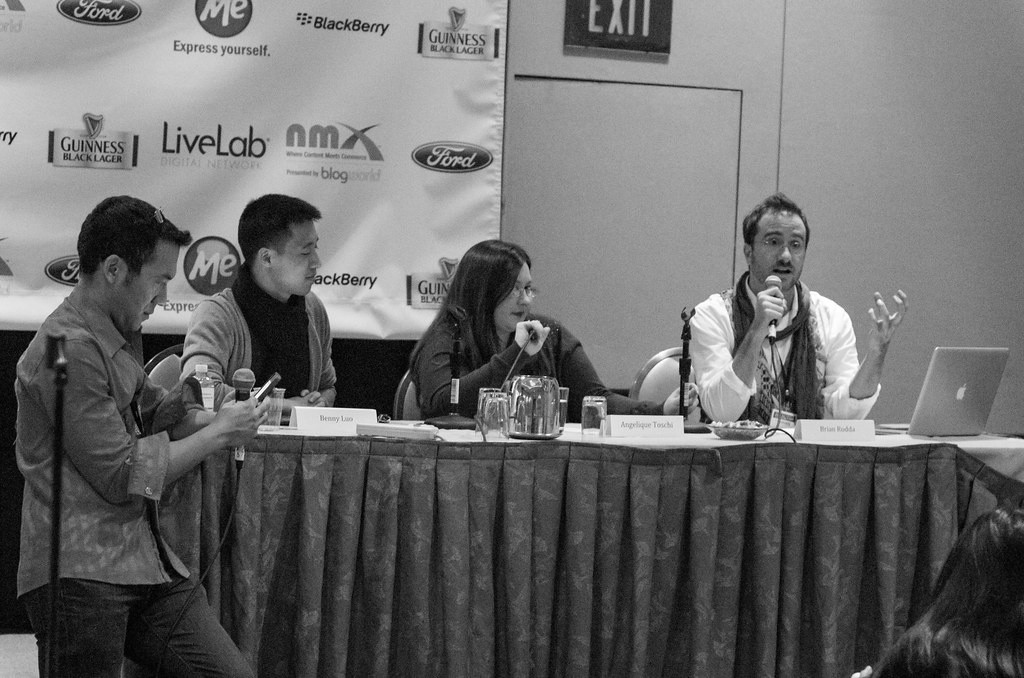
[192,363,216,413]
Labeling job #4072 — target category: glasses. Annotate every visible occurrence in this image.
[507,285,537,298]
[754,239,807,255]
[148,206,170,224]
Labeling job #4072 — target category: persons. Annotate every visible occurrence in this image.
[181,194,336,425]
[411,240,699,431]
[687,193,909,426]
[14,194,271,678]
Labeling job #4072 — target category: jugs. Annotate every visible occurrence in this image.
[503,375,562,440]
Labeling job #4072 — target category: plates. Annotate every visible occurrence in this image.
[705,424,769,440]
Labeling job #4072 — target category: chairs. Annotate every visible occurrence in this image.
[136,344,188,439]
[630,348,699,407]
[392,369,422,422]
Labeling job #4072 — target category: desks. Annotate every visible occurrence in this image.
[207,416,1024,678]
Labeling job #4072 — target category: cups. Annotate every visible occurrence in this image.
[255,388,285,428]
[557,387,569,432]
[476,388,511,439]
[581,396,607,435]
